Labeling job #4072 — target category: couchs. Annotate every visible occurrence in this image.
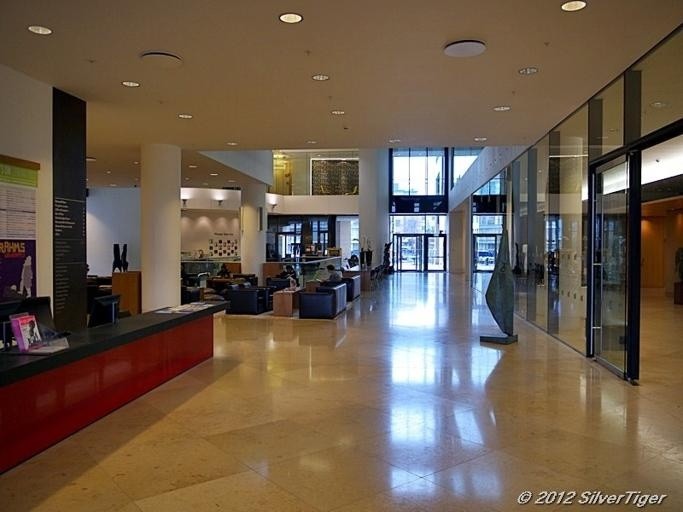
[296,308,349,351]
[221,314,271,344]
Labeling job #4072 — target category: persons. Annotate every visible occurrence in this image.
[25,320,38,347]
[216,265,230,278]
[319,265,341,287]
[285,264,296,278]
[196,250,206,264]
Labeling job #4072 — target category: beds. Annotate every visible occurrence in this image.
[272,316,296,343]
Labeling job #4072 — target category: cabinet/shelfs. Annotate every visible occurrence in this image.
[111,271,141,317]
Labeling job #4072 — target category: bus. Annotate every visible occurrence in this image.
[287,239,359,261]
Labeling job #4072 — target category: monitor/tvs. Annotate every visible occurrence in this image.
[87,294,121,328]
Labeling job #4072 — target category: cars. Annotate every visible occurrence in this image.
[478,249,494,263]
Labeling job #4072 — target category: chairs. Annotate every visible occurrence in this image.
[340,258,385,289]
[181,271,362,320]
